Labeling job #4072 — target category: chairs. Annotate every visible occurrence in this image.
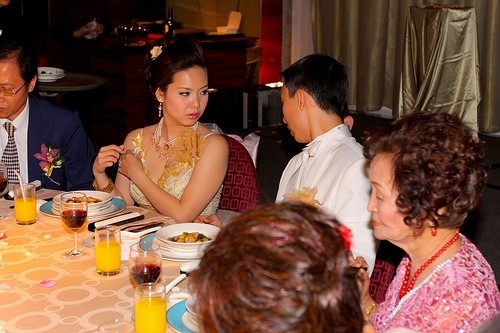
[220,133,261,215]
[369,260,395,305]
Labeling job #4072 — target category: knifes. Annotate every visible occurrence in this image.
[9,197,54,209]
[129,222,165,233]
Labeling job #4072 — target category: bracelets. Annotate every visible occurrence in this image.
[94,177,115,194]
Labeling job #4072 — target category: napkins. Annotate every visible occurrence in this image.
[180,261,200,272]
[35,199,48,213]
[7,179,42,199]
[120,226,161,239]
[94,211,140,228]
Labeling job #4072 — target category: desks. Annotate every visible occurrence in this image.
[393,5,483,137]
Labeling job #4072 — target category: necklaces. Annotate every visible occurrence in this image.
[399,233,459,299]
[153,117,199,157]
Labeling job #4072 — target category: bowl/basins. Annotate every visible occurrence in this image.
[156,222,220,249]
[53,190,112,209]
[185,293,199,317]
[0,178,8,193]
[37,67,64,78]
[121,231,140,260]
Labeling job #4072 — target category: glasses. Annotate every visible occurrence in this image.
[0,82,27,96]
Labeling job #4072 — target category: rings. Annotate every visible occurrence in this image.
[119,162,122,167]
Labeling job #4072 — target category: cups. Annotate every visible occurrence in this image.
[95,224,121,276]
[128,242,163,286]
[134,282,167,333]
[13,183,37,225]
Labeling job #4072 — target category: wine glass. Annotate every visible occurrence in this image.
[116,22,149,46]
[60,192,89,257]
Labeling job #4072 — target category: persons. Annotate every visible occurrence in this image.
[348,111,500,333]
[191,201,365,333]
[0,0,105,70]
[275,53,380,279]
[0,37,97,191]
[92,33,229,224]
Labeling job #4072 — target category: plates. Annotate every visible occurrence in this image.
[166,298,200,333]
[37,74,66,82]
[0,188,9,197]
[152,237,204,254]
[138,232,204,261]
[188,313,199,326]
[40,198,127,218]
[52,201,112,213]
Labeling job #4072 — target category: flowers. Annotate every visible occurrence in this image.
[32,143,66,178]
[334,225,353,252]
[150,45,163,60]
[282,186,322,207]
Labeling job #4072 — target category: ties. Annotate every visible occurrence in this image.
[283,152,310,199]
[1,122,20,184]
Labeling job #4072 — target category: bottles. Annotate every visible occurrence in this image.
[163,7,174,42]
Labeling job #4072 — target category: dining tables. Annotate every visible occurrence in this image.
[0,188,190,333]
